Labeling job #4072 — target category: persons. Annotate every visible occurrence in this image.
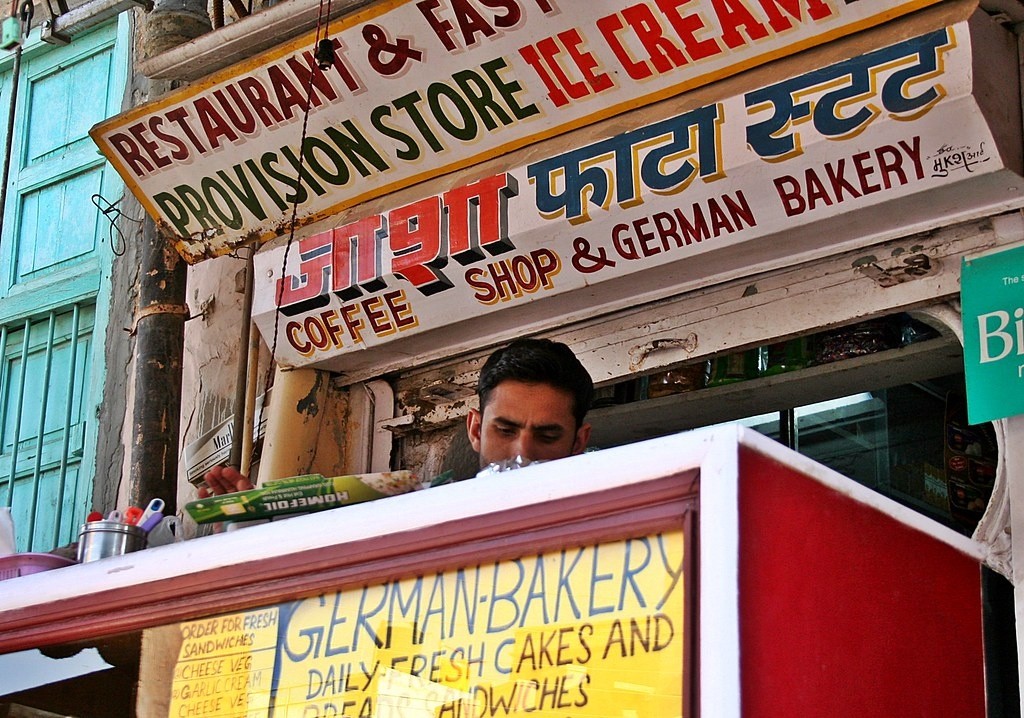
[464,335,598,481]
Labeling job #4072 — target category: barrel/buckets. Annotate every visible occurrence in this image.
[76,521,149,564]
